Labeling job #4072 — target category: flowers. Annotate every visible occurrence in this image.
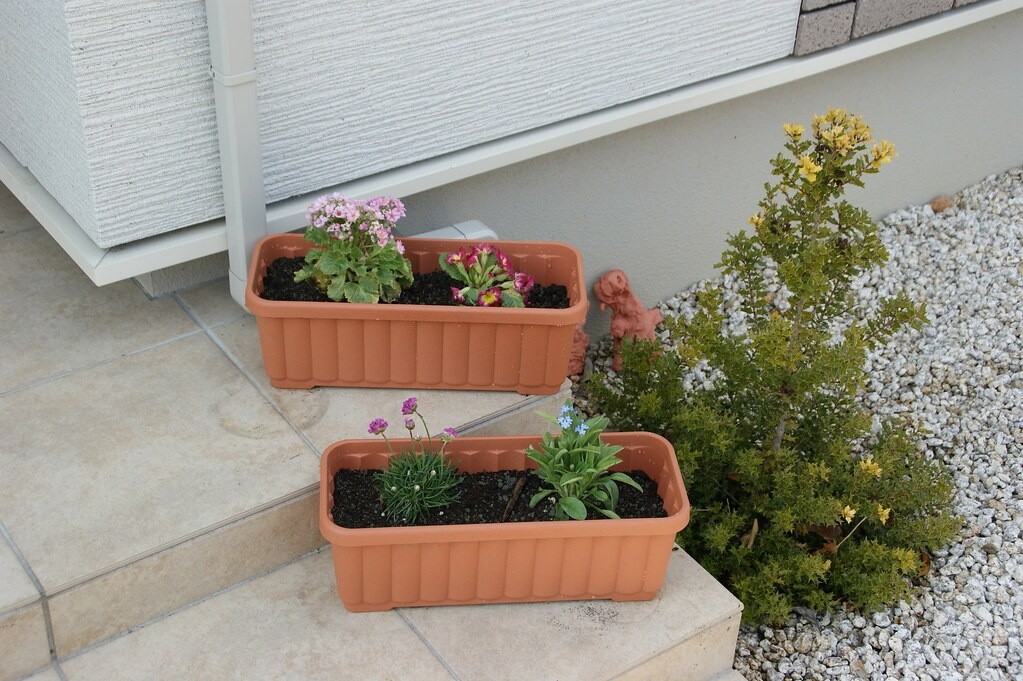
[366,397,645,524]
[293,189,537,308]
[577,105,969,630]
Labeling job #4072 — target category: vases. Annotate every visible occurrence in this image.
[318,431,691,614]
[244,232,588,397]
